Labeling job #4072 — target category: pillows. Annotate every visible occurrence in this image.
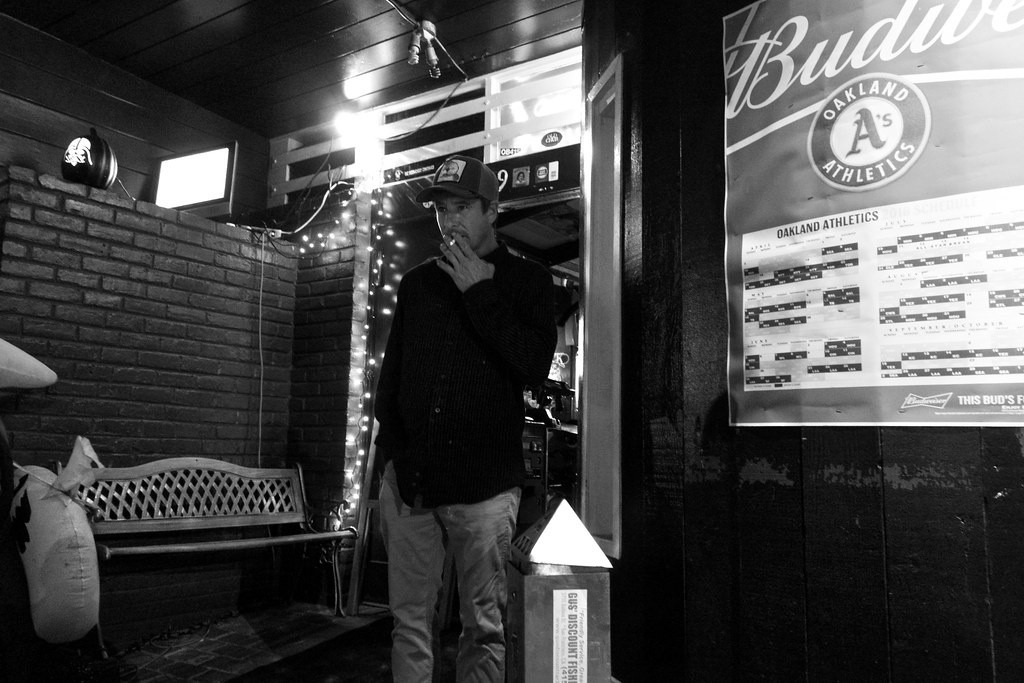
[0,337,58,389]
[9,465,101,645]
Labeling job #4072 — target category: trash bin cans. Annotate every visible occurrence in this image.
[504,498,614,683]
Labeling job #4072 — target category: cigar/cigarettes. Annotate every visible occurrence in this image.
[450,239,455,246]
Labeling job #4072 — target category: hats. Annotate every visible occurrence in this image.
[416,155,499,204]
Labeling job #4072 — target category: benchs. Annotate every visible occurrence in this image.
[54,457,361,662]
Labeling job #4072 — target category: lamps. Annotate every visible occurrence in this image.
[405,32,422,65]
[425,43,442,79]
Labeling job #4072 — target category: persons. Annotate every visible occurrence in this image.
[374,155,558,683]
